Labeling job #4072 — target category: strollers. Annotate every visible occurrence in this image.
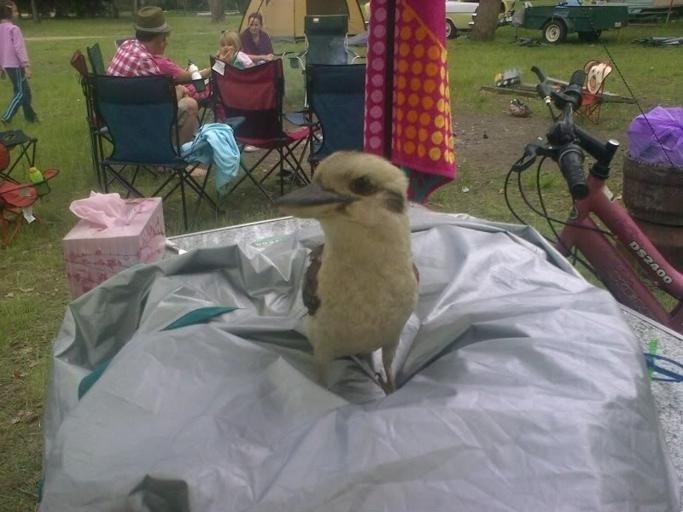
[1,130,59,247]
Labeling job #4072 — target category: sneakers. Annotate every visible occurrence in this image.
[179,166,208,176]
[245,145,260,152]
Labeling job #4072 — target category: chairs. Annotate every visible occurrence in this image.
[86,41,106,75]
[79,72,247,232]
[66,47,176,187]
[291,60,369,185]
[193,53,308,214]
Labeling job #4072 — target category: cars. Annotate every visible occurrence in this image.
[445,1,478,38]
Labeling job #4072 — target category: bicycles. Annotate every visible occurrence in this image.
[505,65,683,330]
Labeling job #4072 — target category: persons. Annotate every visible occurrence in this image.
[106,6,209,176]
[0,3,38,124]
[219,32,253,67]
[238,13,273,64]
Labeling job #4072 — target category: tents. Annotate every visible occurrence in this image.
[239,1,368,41]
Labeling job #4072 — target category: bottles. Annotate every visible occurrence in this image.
[31,167,49,195]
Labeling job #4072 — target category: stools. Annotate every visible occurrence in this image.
[2,130,37,187]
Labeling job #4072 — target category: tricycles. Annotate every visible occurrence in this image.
[498,0,628,44]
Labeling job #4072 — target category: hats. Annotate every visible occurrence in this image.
[132,5,175,37]
